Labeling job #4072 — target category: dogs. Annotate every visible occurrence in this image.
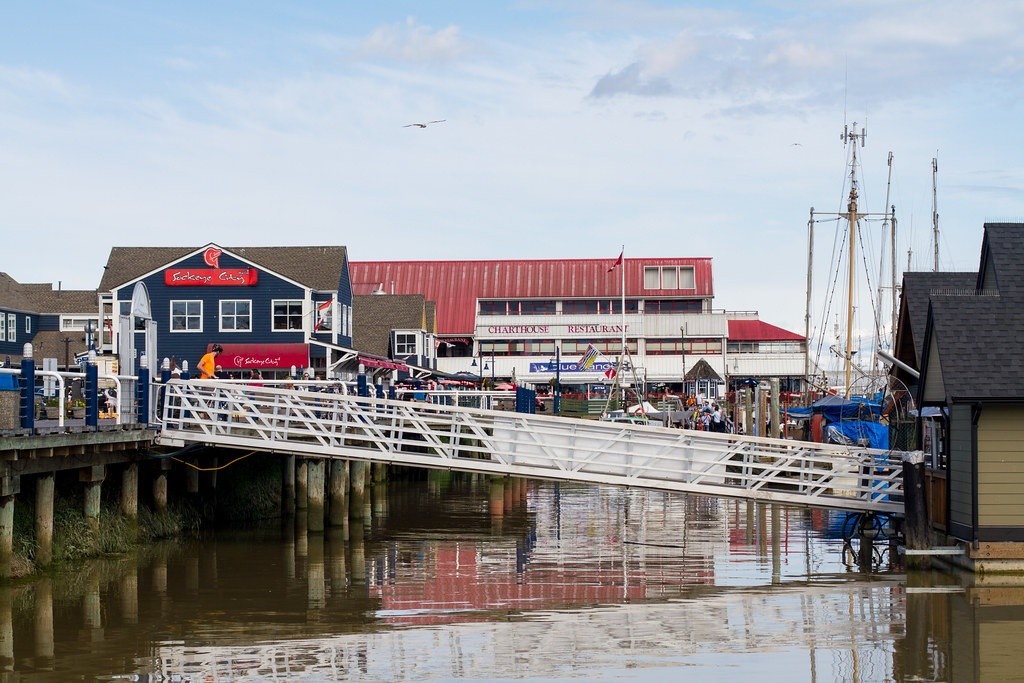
[96,386,118,416]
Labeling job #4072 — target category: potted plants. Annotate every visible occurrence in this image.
[43,399,69,420]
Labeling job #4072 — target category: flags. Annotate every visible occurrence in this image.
[577,345,600,373]
[314,298,334,331]
[607,252,623,272]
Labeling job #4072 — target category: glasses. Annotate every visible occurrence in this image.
[304,376,308,377]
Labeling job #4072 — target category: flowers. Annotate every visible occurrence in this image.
[71,399,85,407]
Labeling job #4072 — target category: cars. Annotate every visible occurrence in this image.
[34,386,117,413]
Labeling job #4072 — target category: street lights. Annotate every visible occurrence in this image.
[471,343,482,391]
[484,349,495,391]
[548,346,560,413]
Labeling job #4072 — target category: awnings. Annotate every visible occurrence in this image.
[357,356,408,372]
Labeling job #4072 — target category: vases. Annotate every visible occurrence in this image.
[70,407,85,419]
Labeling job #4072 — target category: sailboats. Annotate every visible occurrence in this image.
[779,122,903,520]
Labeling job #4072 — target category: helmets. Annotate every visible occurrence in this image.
[212,344,223,352]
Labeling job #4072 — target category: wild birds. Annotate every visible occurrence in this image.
[402,120,447,128]
[793,143,802,147]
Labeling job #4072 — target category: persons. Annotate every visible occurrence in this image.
[197,344,810,441]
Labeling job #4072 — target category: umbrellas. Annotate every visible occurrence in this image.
[450,371,480,390]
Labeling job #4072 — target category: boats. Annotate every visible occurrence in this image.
[598,334,649,425]
